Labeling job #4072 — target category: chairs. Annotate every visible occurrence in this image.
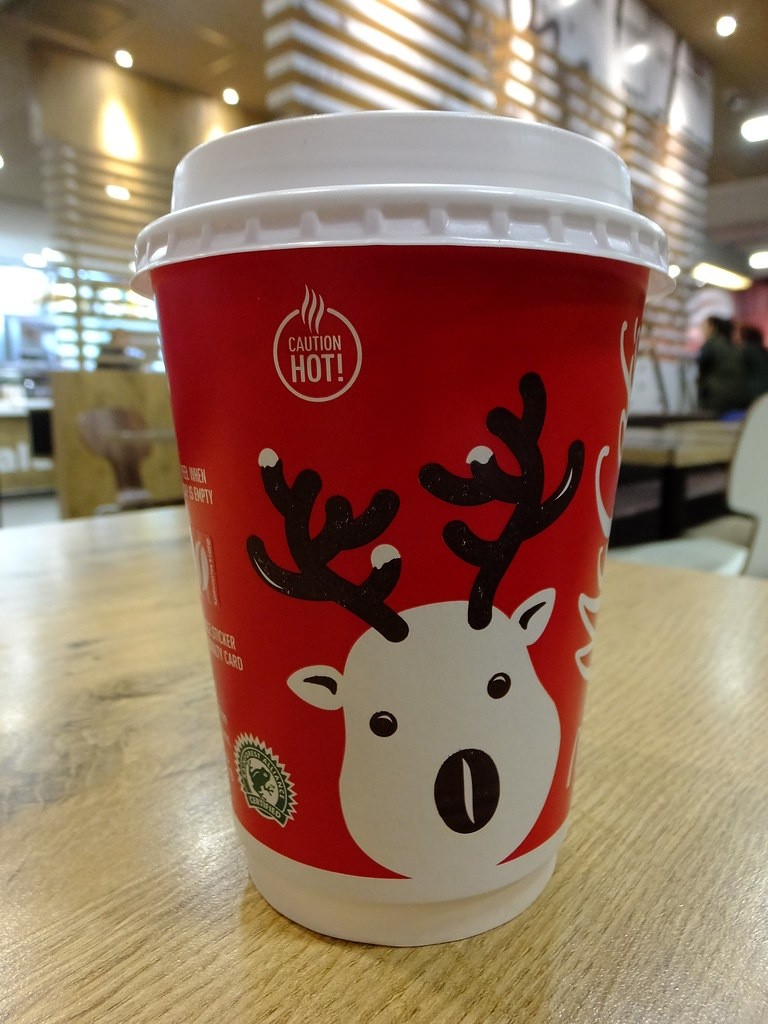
[27,408,54,478]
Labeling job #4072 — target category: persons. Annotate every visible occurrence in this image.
[97,327,158,370]
[20,326,52,386]
[695,316,768,421]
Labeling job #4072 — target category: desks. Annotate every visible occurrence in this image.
[0,411,56,499]
[0,505,768,1024]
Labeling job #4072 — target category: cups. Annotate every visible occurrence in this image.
[129,111,676,947]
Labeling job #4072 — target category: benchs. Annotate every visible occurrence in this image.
[622,417,742,546]
